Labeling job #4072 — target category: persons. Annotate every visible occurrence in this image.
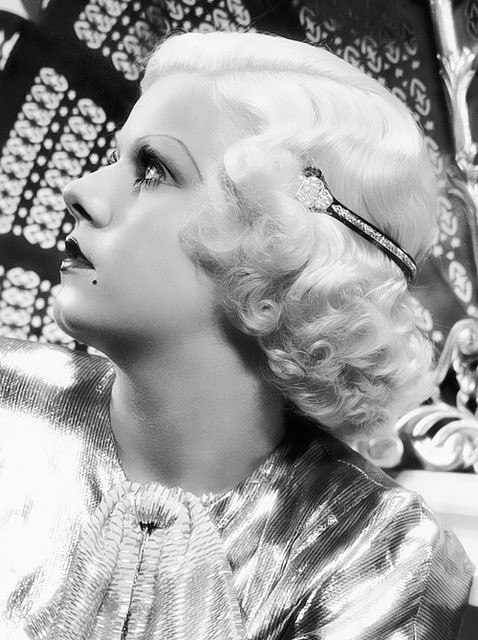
[0,29,478,637]
